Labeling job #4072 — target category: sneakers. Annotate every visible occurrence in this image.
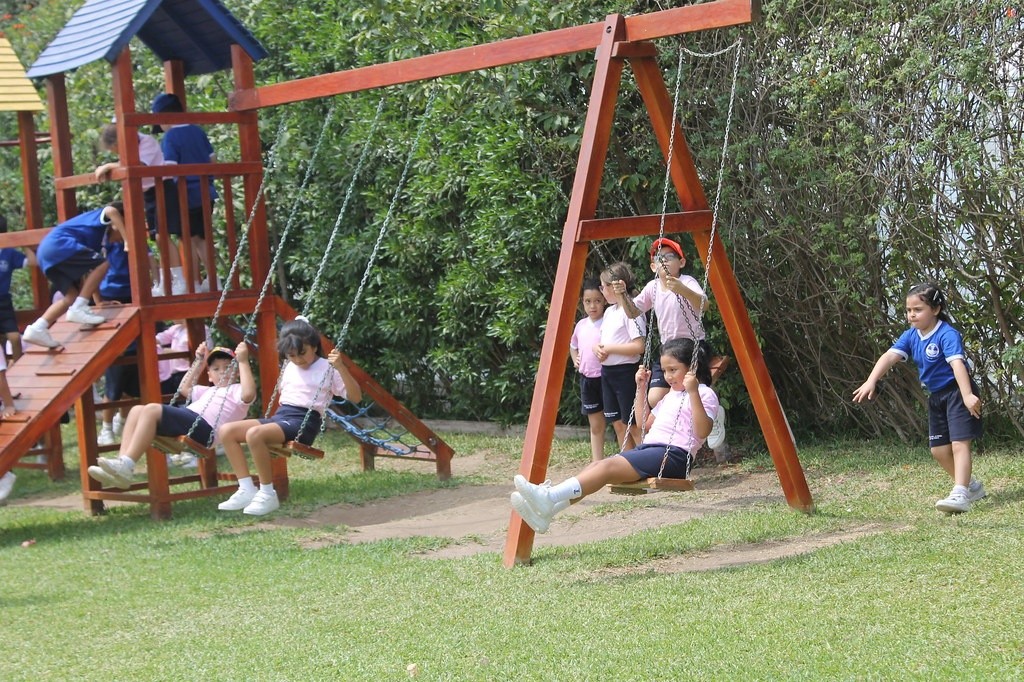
[22,325,60,347]
[511,492,549,534]
[0,473,17,500]
[97,457,133,483]
[66,306,105,324]
[514,475,555,520]
[243,491,279,515]
[96,430,115,444]
[935,489,970,512]
[88,465,129,489]
[218,486,258,510]
[112,416,124,436]
[968,484,985,500]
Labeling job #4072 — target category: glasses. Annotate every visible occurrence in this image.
[654,252,680,263]
[599,285,611,292]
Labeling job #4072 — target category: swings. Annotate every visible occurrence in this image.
[605,34,746,495]
[482,55,731,388]
[262,75,443,460]
[148,94,337,461]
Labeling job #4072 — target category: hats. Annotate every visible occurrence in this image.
[650,238,683,258]
[152,93,182,133]
[206,346,236,365]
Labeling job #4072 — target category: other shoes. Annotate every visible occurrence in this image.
[170,278,186,295]
[201,279,223,291]
[37,444,48,464]
[151,280,165,297]
[194,283,202,294]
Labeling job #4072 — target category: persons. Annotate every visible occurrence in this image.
[612,238,725,449]
[853,284,986,513]
[593,263,648,451]
[94,94,224,297]
[218,316,362,516]
[21,202,226,468]
[570,279,607,462]
[511,338,719,534]
[0,213,70,501]
[88,341,257,488]
